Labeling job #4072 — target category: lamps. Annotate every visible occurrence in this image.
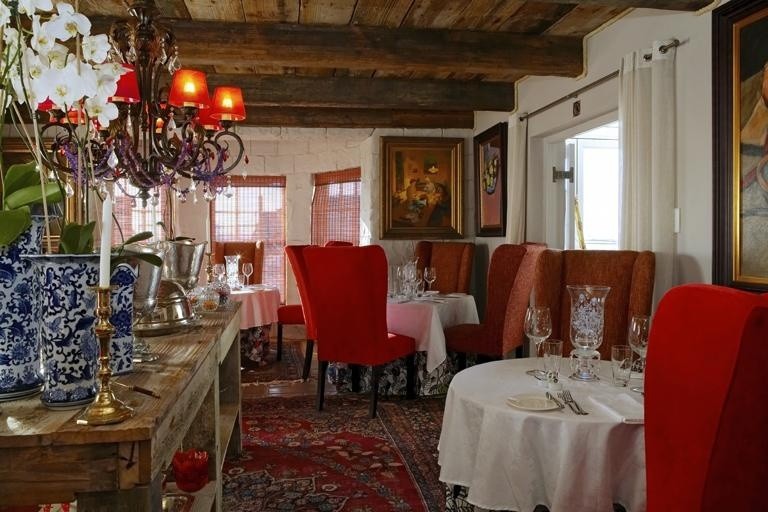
[38,0,249,208]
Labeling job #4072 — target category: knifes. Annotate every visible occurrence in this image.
[546,391,564,409]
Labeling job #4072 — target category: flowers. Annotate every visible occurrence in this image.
[1,0,128,246]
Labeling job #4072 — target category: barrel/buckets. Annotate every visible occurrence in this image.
[156,240,208,291]
[114,243,164,326]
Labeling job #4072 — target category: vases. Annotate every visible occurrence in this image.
[100,251,140,375]
[20,253,100,409]
[0,214,58,402]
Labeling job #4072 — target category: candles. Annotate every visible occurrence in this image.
[172,449,210,491]
[99,192,112,288]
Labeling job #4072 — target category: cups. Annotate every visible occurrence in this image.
[543,338,563,378]
[611,345,633,387]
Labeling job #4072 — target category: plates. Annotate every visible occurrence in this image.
[507,393,564,411]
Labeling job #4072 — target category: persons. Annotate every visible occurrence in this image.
[424,177,437,194]
[406,178,417,203]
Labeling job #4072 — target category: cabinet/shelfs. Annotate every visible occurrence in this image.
[1,298,243,510]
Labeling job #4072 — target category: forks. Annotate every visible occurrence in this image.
[557,392,580,416]
[565,391,588,415]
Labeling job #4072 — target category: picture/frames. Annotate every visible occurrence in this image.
[474,121,508,237]
[379,135,465,239]
[711,0,768,289]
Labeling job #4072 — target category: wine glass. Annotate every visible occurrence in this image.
[242,263,253,288]
[215,263,225,280]
[397,264,435,297]
[628,316,652,393]
[524,306,552,375]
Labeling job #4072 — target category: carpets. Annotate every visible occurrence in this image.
[241,339,312,385]
[221,393,445,512]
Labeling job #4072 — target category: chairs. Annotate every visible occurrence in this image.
[210,241,263,284]
